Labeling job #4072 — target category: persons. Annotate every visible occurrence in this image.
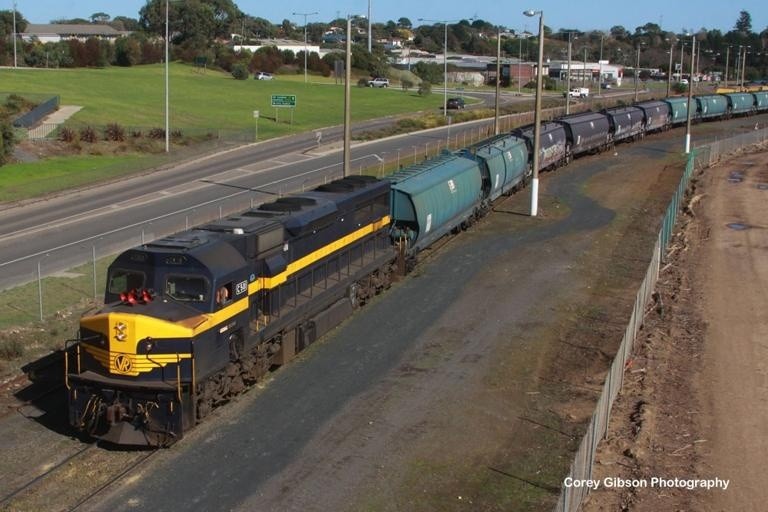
[216,286,228,303]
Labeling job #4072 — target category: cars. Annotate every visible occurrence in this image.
[367,77,389,88]
[254,72,274,81]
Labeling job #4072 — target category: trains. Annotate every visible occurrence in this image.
[64,91,768,448]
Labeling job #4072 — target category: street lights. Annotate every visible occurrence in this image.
[416,17,473,116]
[292,11,320,81]
[342,13,365,177]
[447,97,466,109]
